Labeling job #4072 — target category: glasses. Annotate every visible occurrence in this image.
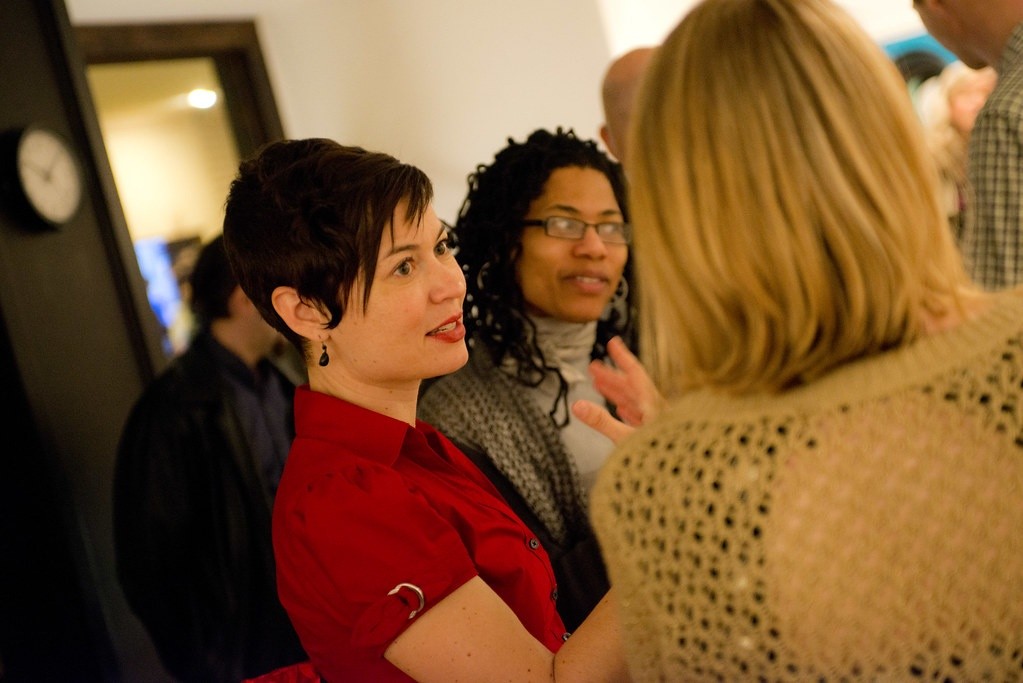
[527,215,632,244]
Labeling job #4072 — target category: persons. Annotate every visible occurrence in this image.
[417,128,659,636]
[219,136,669,683]
[892,2,1023,293]
[113,236,309,682]
[587,0,1023,683]
[598,48,658,164]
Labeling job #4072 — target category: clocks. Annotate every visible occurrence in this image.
[0,124,92,236]
